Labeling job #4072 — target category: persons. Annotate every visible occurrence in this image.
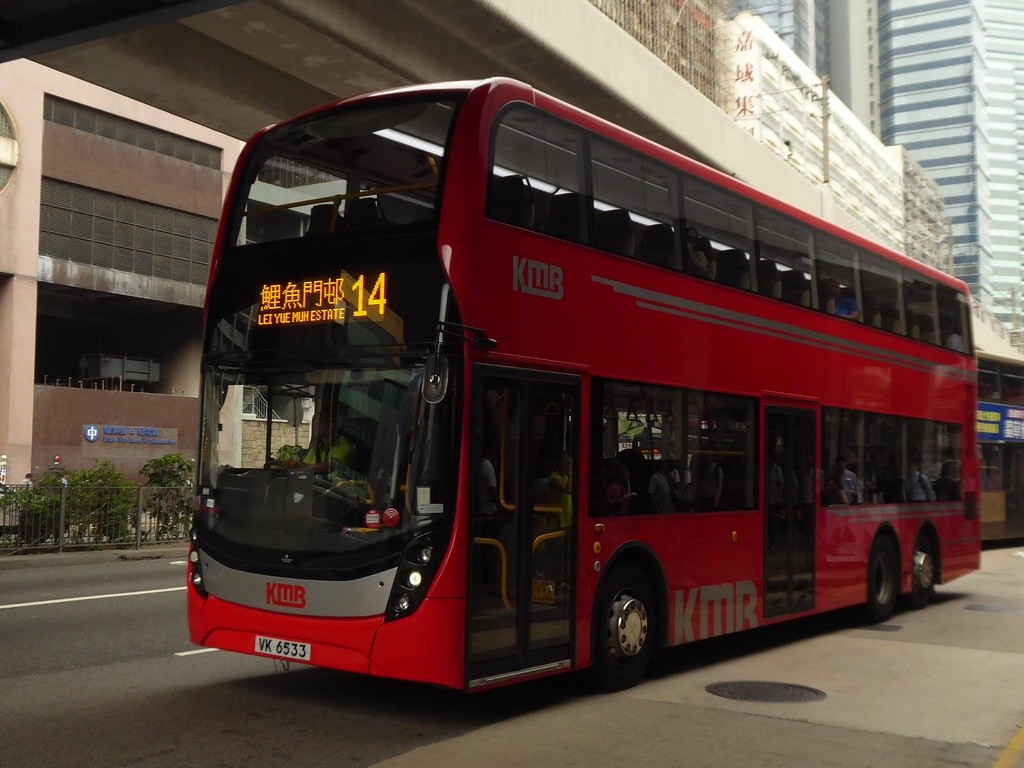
[826,444,865,506]
[822,278,861,321]
[684,227,709,277]
[940,316,956,348]
[941,461,962,501]
[911,457,938,503]
[284,409,353,493]
[21,473,36,485]
[523,407,573,589]
[478,437,499,585]
[56,472,70,486]
[604,455,694,514]
[698,451,723,511]
[766,453,812,553]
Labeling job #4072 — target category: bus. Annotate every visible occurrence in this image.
[957,349,1023,547]
[184,78,981,694]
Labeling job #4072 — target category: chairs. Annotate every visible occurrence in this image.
[306,170,962,514]
